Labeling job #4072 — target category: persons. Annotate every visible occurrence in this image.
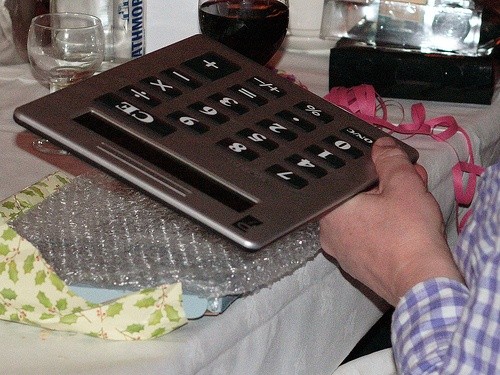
[318,136,499,375]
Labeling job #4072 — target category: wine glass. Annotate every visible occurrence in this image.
[29,11,107,157]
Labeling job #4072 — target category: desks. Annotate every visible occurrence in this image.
[0,37,500,375]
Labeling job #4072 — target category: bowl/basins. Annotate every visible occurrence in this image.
[198,1,291,70]
